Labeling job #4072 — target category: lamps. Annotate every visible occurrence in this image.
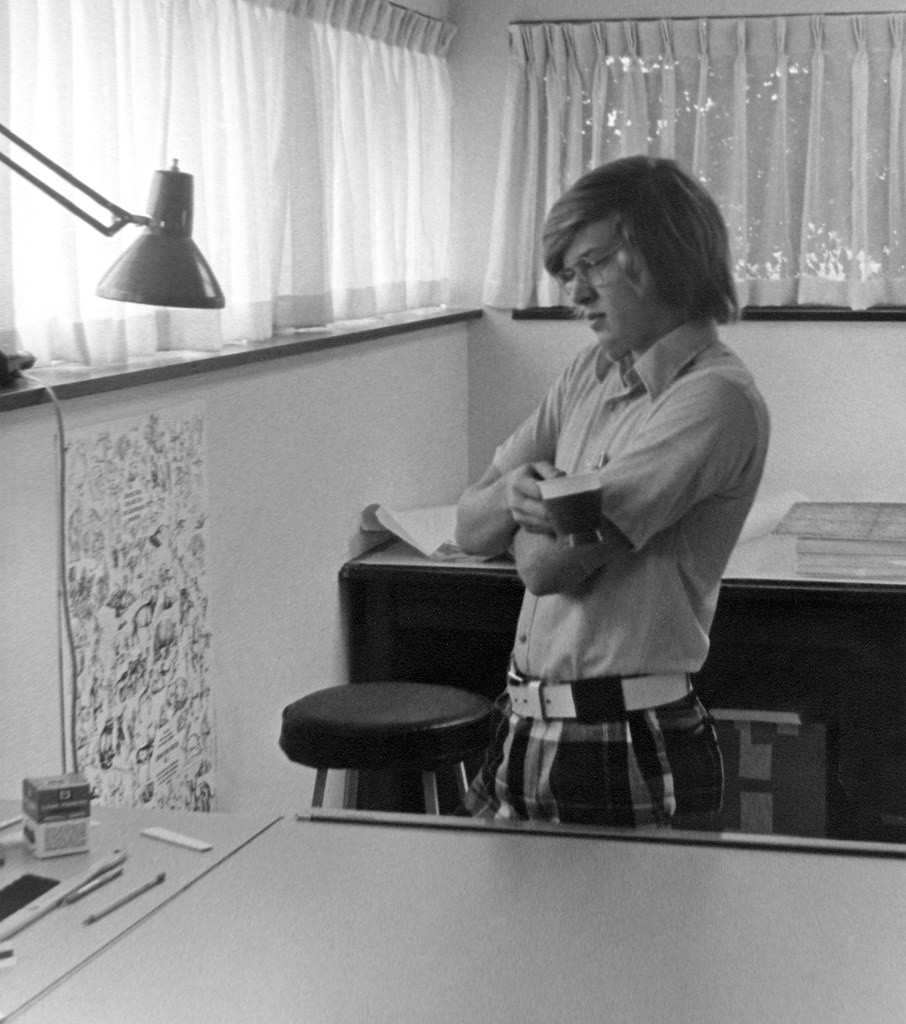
[0,124,227,310]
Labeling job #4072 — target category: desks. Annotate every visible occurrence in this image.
[0,796,905,1023]
[341,525,906,844]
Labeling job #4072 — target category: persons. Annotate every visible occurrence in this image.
[455,155,771,830]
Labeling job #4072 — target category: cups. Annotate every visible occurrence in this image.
[537,470,604,547]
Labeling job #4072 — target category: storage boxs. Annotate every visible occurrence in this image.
[713,712,842,836]
[23,778,91,859]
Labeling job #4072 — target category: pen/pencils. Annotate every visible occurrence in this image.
[66,869,121,904]
[82,871,164,925]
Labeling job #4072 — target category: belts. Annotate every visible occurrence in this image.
[505,663,695,719]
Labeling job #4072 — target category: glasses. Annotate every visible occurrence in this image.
[550,241,628,297]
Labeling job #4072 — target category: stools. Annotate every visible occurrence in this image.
[279,678,499,816]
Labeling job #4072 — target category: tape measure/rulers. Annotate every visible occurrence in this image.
[140,827,213,852]
